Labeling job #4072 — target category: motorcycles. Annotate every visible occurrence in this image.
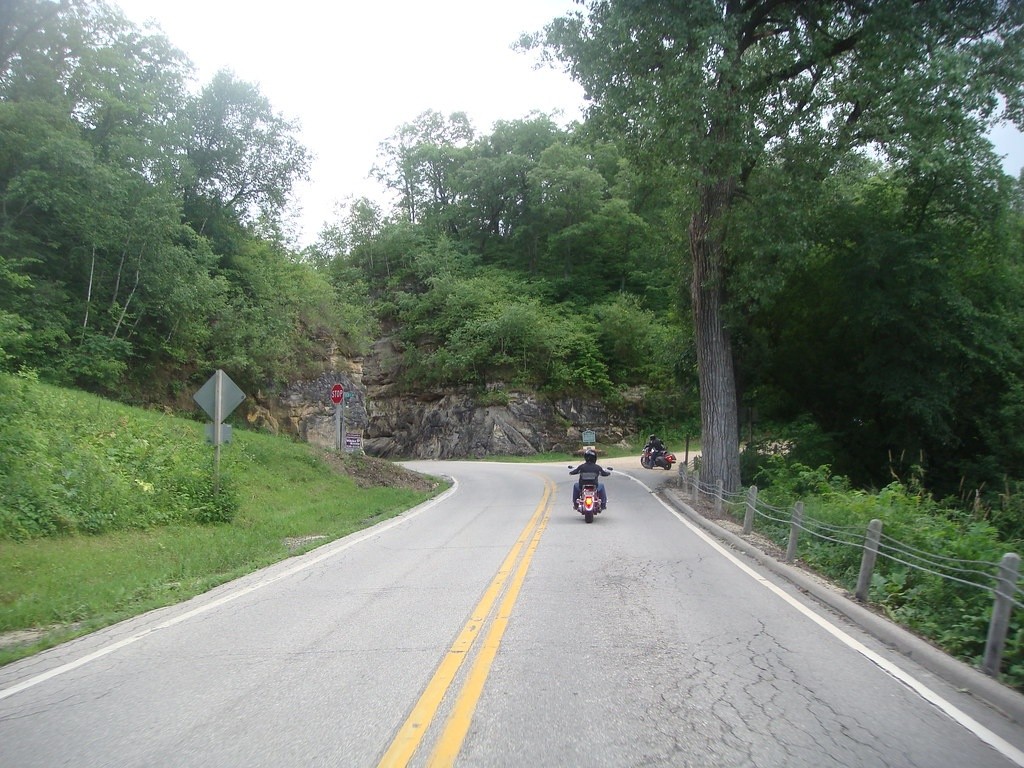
[640,444,677,470]
[568,465,614,523]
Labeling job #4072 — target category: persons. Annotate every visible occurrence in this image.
[569,450,611,510]
[646,434,664,468]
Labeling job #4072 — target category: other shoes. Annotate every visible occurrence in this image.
[601,503,606,509]
[573,503,579,510]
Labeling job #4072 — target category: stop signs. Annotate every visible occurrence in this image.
[330,384,343,404]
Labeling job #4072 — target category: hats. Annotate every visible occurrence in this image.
[650,433,655,437]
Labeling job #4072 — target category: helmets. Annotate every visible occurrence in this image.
[584,449,597,463]
